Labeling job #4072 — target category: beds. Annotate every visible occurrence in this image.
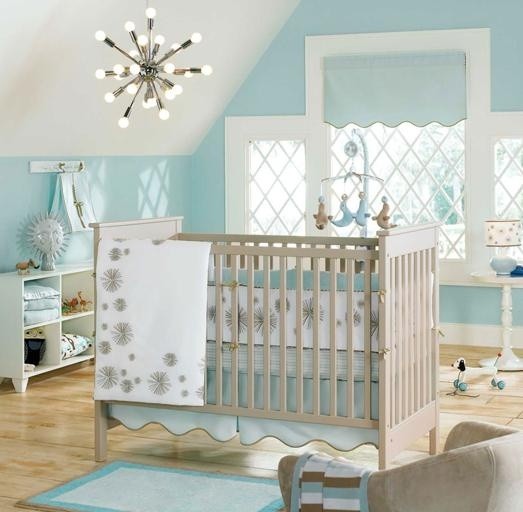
[88,215,444,469]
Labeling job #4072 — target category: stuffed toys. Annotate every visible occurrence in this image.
[61,289,91,317]
[15,258,39,275]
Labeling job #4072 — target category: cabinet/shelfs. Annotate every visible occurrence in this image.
[1,262,93,394]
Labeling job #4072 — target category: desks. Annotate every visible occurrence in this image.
[470,270,522,371]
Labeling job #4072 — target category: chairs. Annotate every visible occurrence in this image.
[279,421,522,512]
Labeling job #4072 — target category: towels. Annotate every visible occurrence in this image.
[95,239,215,408]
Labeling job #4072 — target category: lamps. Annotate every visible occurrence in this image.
[94,0,213,128]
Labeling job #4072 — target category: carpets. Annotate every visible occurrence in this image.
[16,459,284,511]
